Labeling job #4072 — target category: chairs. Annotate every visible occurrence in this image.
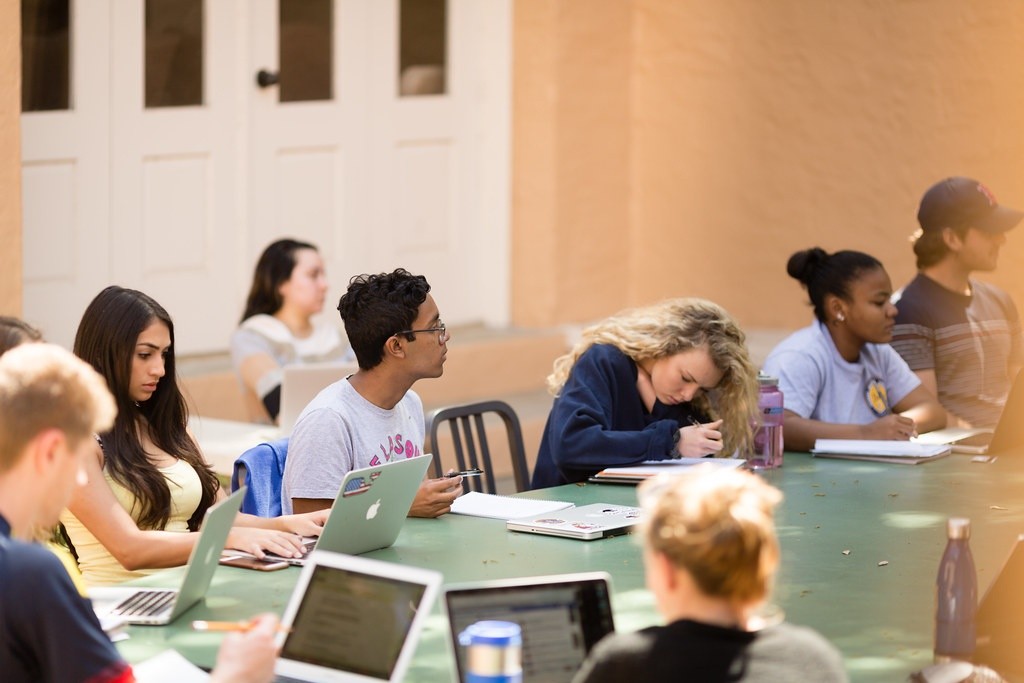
[422,398,532,496]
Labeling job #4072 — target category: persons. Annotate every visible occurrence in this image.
[228,237,356,428]
[890,177,1024,431]
[530,298,755,491]
[570,463,848,683]
[759,247,948,451]
[58,285,330,587]
[280,270,463,519]
[0,316,280,682]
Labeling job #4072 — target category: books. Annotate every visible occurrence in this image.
[593,463,746,485]
[812,439,954,466]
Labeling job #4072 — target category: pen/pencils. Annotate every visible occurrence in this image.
[687,414,702,428]
[191,620,294,634]
[444,468,484,478]
[888,406,899,415]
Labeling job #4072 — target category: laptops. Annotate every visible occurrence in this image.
[82,486,247,626]
[442,572,618,683]
[220,452,433,566]
[506,502,642,540]
[275,551,444,683]
[901,366,1024,455]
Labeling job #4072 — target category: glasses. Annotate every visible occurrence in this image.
[393,319,446,344]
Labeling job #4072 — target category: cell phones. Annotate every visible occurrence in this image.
[218,555,289,571]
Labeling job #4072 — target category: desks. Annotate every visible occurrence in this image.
[185,414,289,492]
[120,452,1024,683]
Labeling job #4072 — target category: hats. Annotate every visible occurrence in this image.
[918,176,1024,231]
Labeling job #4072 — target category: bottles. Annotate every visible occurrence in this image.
[750,376,784,469]
[933,518,977,664]
[458,620,524,683]
